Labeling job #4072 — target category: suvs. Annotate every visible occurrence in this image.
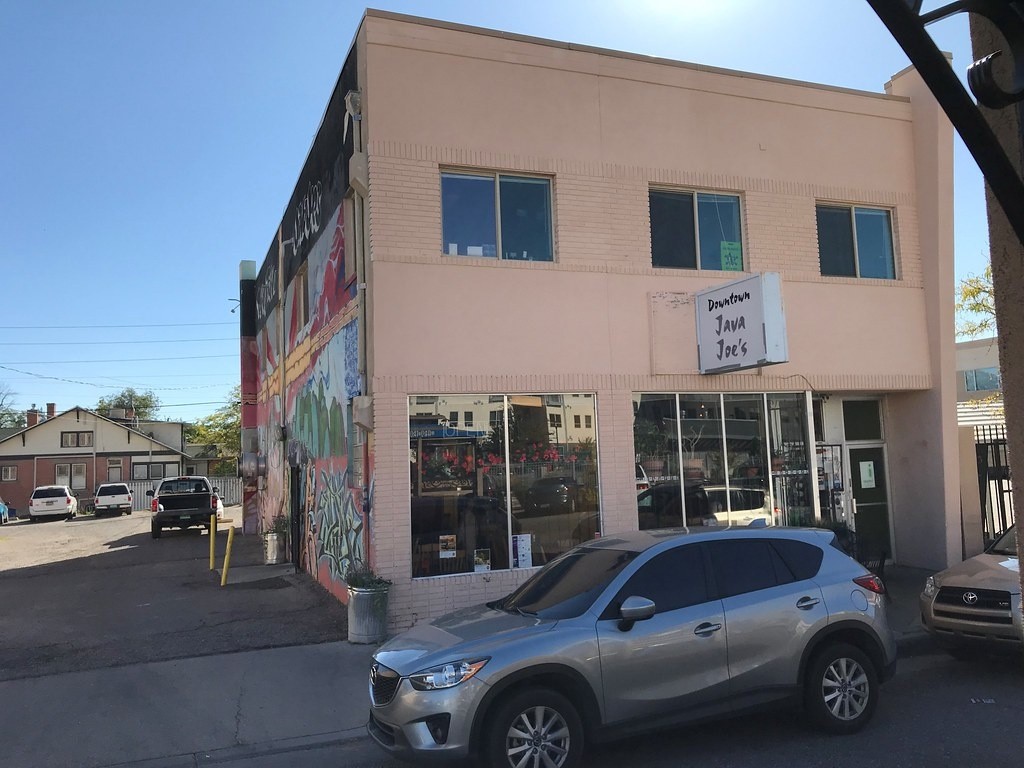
[472,465,782,527]
[366,526,897,768]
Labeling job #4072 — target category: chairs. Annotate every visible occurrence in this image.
[439,550,470,572]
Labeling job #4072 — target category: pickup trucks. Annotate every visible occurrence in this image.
[145,476,219,539]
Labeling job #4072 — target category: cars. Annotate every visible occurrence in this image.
[29,486,78,521]
[919,523,1024,650]
[0,497,8,525]
[92,482,134,516]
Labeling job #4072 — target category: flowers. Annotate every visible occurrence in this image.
[412,436,596,479]
